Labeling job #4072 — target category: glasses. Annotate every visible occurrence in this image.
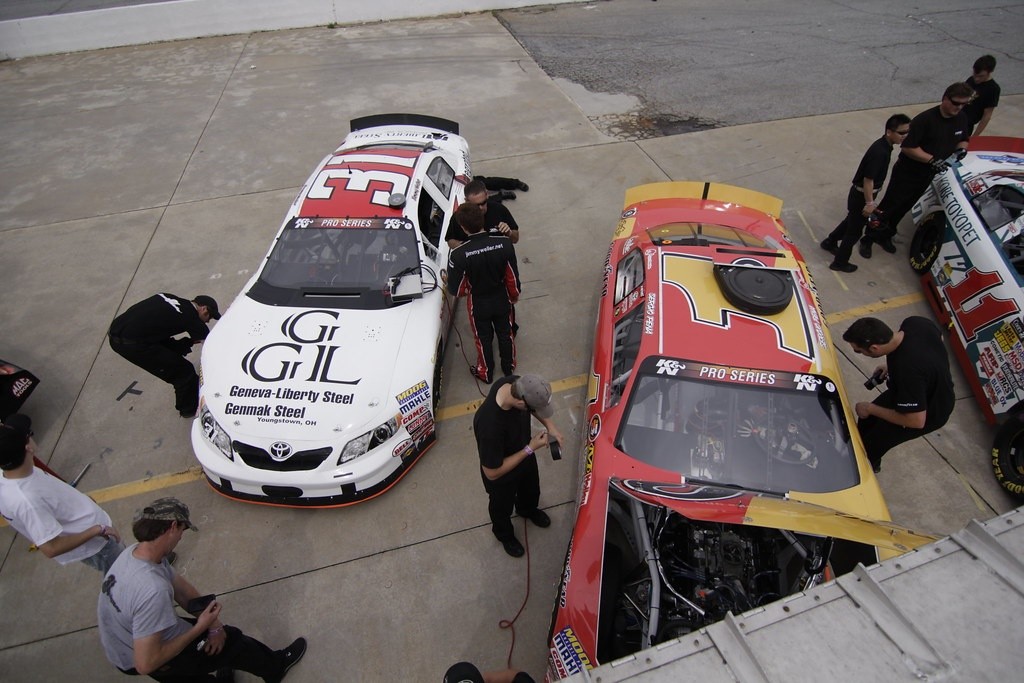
[478,199,487,206]
[28,430,34,438]
[894,129,910,135]
[949,96,972,106]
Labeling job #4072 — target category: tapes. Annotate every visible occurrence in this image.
[550,440,562,461]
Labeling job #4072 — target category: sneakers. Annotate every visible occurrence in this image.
[860,236,872,258]
[497,532,525,557]
[877,238,896,253]
[264,637,307,683]
[515,502,551,528]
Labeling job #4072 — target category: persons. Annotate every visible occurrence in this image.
[472,174,529,201]
[859,81,973,258]
[0,414,177,574]
[821,112,912,272]
[446,181,520,339]
[446,203,522,383]
[97,496,307,683]
[964,54,1001,137]
[473,375,564,557]
[108,291,222,419]
[843,316,956,472]
[443,662,536,683]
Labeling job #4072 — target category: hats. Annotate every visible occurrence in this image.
[442,662,484,683]
[0,413,32,464]
[141,497,198,533]
[517,374,554,419]
[191,295,221,321]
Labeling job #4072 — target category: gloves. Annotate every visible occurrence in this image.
[930,158,951,172]
[956,148,967,162]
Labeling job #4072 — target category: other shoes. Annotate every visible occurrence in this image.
[500,189,516,200]
[180,409,197,418]
[470,365,494,385]
[820,239,839,256]
[830,263,857,273]
[518,181,529,192]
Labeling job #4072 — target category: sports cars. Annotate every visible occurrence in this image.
[908,132,1024,508]
[190,110,471,510]
[545,181,894,683]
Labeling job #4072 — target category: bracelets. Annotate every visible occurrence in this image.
[98,524,106,535]
[208,623,224,636]
[509,229,512,236]
[866,201,874,205]
[525,445,534,455]
